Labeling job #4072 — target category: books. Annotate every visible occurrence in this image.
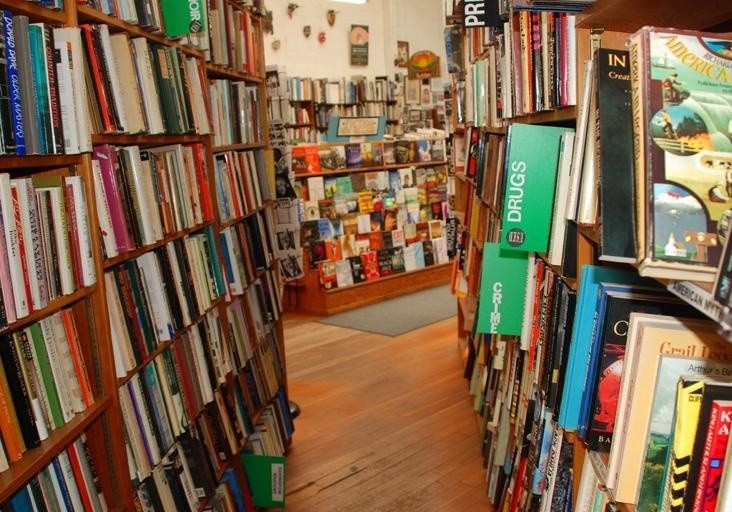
[0,0,297,327]
[1,207,297,511]
[447,2,731,512]
[297,60,444,290]
